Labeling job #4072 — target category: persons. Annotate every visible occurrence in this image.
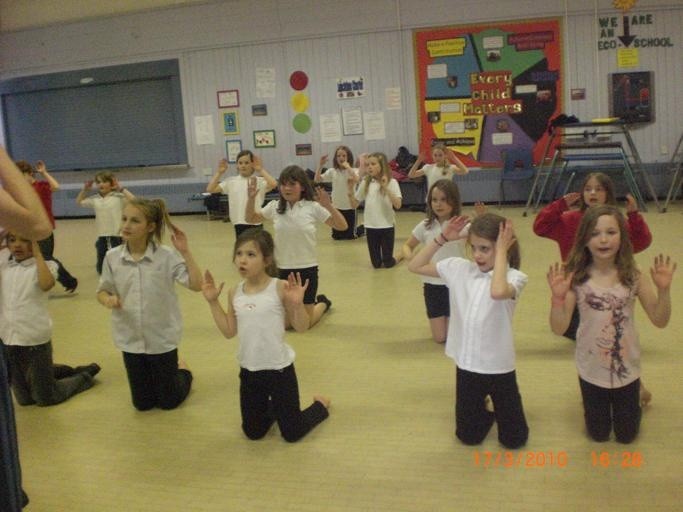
[15,159,78,293]
[77,170,137,274]
[409,214,528,450]
[406,145,469,206]
[401,180,472,345]
[1,145,52,512]
[97,197,203,410]
[536,173,652,340]
[314,145,366,240]
[1,228,101,407]
[546,205,677,445]
[244,165,347,331]
[203,228,331,443]
[347,153,404,269]
[206,150,279,242]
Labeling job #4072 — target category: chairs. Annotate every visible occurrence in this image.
[498,144,535,207]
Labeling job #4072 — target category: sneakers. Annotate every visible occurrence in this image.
[75,361,101,377]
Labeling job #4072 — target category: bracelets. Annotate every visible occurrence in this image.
[120,188,124,192]
[552,298,565,306]
[432,234,448,247]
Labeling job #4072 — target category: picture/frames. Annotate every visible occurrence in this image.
[253,130,275,147]
[611,71,651,124]
[226,140,242,164]
[221,111,240,135]
[217,90,240,109]
[251,104,267,116]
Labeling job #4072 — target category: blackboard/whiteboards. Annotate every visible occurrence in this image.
[0,57,192,173]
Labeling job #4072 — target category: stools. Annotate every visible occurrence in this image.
[523,117,660,217]
[550,153,641,214]
[560,165,637,210]
[535,142,647,218]
